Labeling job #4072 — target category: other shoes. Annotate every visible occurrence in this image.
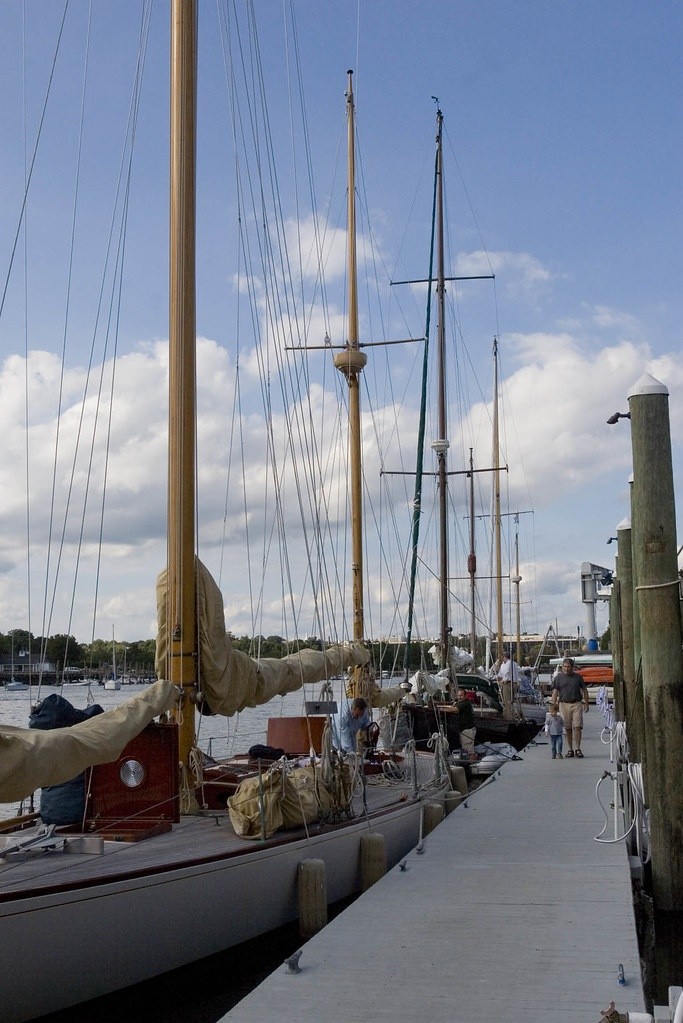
[552,754,556,759]
[557,753,563,759]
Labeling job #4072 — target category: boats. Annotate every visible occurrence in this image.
[330,673,350,680]
[62,677,102,687]
[376,671,389,679]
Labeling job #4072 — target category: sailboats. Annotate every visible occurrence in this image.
[0,0,454,1023]
[388,108,552,754]
[104,626,123,690]
[4,633,29,691]
[117,646,157,685]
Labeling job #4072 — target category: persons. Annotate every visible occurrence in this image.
[332,697,371,758]
[490,651,519,718]
[552,659,589,758]
[545,705,564,759]
[453,689,476,759]
[519,670,538,704]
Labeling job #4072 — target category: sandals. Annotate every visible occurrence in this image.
[565,750,574,758]
[575,749,583,758]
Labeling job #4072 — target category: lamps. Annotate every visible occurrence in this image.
[606,536,618,545]
[606,412,631,424]
